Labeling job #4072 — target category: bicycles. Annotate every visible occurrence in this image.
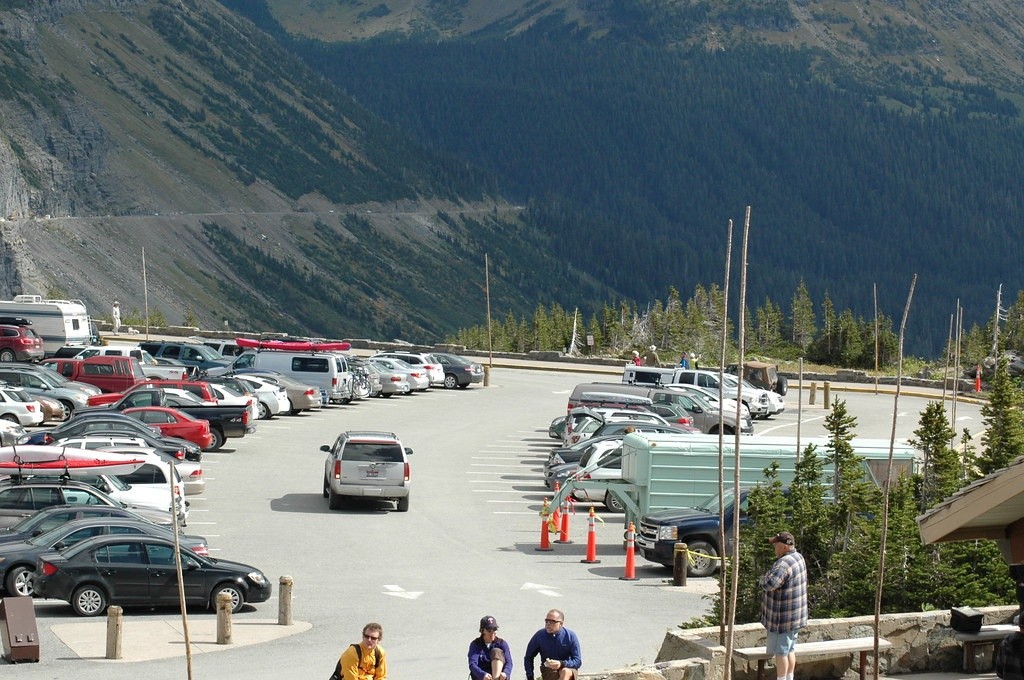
[348,364,372,397]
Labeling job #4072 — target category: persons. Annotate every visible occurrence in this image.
[340,623,387,680]
[112,300,121,337]
[523,609,582,680]
[468,615,513,680]
[631,345,702,370]
[758,531,808,680]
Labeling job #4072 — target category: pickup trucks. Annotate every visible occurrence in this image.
[70,389,257,452]
[52,346,189,381]
[37,356,145,393]
[637,486,873,577]
[567,371,784,435]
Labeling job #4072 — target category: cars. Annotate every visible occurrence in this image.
[190,369,322,420]
[348,350,485,398]
[0,362,212,496]
[0,505,271,617]
[543,434,625,490]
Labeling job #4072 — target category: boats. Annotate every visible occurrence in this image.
[235,337,350,352]
[0,444,147,479]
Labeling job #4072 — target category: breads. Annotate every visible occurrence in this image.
[546,658,555,665]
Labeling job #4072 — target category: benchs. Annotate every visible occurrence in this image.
[953,624,1021,674]
[732,638,892,680]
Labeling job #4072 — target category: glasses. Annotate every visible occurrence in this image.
[545,619,561,624]
[486,629,497,632]
[363,633,379,640]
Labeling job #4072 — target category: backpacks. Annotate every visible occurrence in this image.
[329,644,380,680]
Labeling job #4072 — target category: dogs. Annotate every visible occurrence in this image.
[127,327,139,335]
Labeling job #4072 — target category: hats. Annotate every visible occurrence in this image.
[650,345,657,351]
[113,301,121,306]
[769,532,795,545]
[690,353,695,357]
[481,616,499,627]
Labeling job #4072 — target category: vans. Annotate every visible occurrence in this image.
[207,350,350,402]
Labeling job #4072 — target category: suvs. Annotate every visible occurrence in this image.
[1,476,179,531]
[572,439,624,513]
[724,362,788,395]
[570,408,695,435]
[0,318,44,362]
[319,430,413,514]
[26,462,189,525]
[562,403,694,448]
[191,336,255,364]
[139,340,230,375]
